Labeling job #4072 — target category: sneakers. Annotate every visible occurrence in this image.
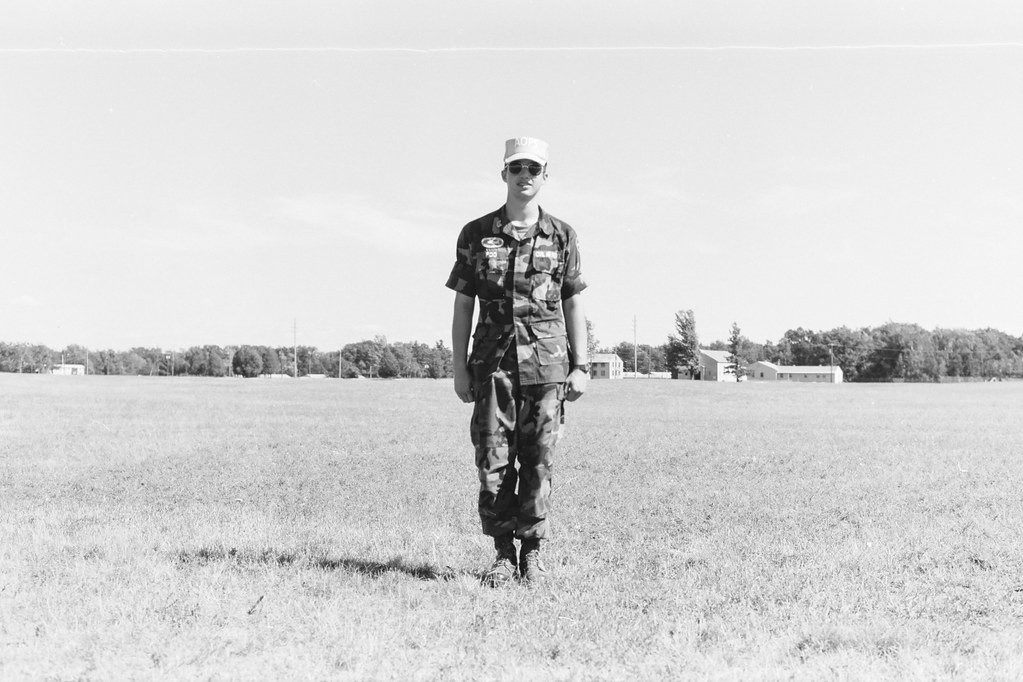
[486,553,517,587]
[520,549,546,585]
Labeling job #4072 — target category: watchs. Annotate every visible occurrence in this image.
[574,364,590,372]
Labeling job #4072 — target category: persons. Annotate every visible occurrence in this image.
[445,136,590,588]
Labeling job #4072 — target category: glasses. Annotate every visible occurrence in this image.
[504,162,542,175]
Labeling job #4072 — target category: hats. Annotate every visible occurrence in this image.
[504,138,549,166]
